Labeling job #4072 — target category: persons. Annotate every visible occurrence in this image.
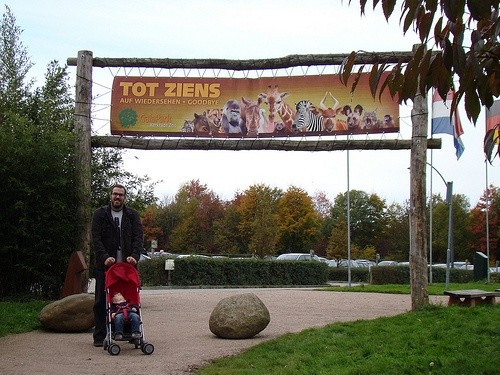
[91,185,145,346]
[110,290,140,339]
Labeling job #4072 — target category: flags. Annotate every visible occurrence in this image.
[486,100,500,162]
[431,77,465,160]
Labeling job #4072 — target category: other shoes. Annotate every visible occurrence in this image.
[132,332,140,339]
[115,334,122,341]
[94,336,103,346]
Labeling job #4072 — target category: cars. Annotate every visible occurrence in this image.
[146,252,500,273]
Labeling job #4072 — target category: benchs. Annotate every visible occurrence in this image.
[444,289,500,308]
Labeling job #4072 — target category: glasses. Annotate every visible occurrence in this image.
[113,193,123,196]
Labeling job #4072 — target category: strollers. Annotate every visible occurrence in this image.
[104,261,154,356]
